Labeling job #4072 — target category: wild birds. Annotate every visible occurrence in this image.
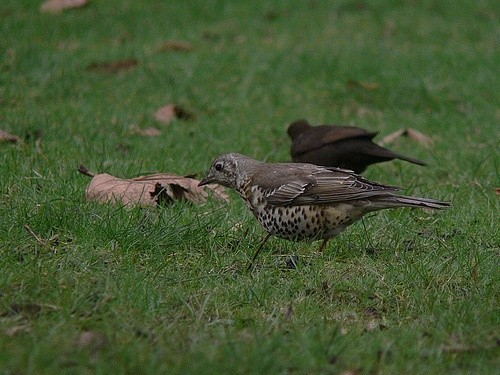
[286,118,428,176]
[197,151,452,257]
